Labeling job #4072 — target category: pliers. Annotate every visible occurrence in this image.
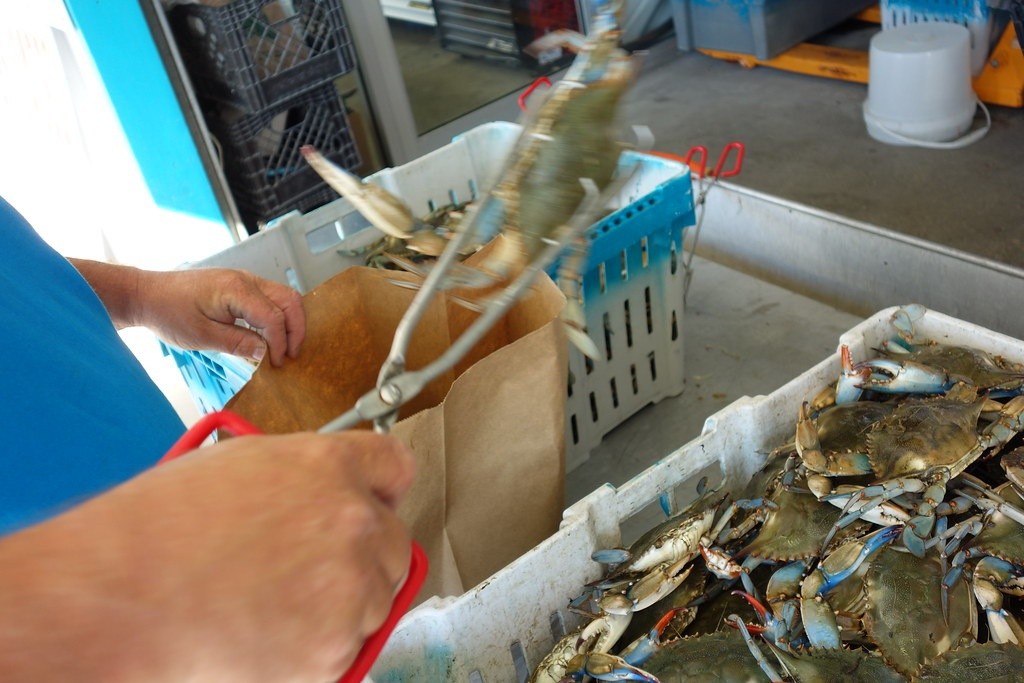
[151,72,663,683]
[684,141,745,307]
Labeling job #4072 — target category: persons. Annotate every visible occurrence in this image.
[0,195,420,683]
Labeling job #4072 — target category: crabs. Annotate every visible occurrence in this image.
[524,336,1024,682]
[296,0,635,363]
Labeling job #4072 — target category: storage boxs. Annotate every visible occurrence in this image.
[156,118,696,476]
[670,0,875,61]
[166,0,359,234]
[880,0,994,76]
[361,302,1024,683]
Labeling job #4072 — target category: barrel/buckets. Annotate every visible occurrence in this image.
[863,21,990,148]
